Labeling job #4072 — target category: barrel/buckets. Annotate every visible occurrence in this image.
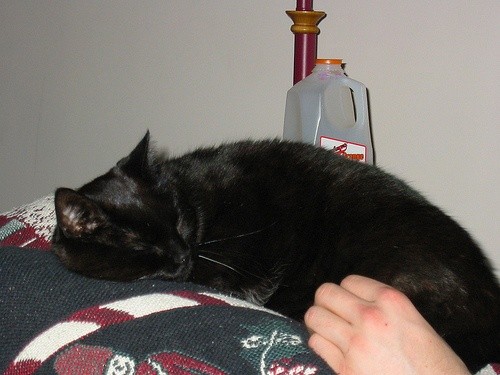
[282,59,373,166]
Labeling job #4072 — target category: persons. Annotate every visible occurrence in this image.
[303,273,473,375]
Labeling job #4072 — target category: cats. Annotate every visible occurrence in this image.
[53,128,500,374]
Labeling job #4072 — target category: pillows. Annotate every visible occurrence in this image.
[1,244,338,374]
